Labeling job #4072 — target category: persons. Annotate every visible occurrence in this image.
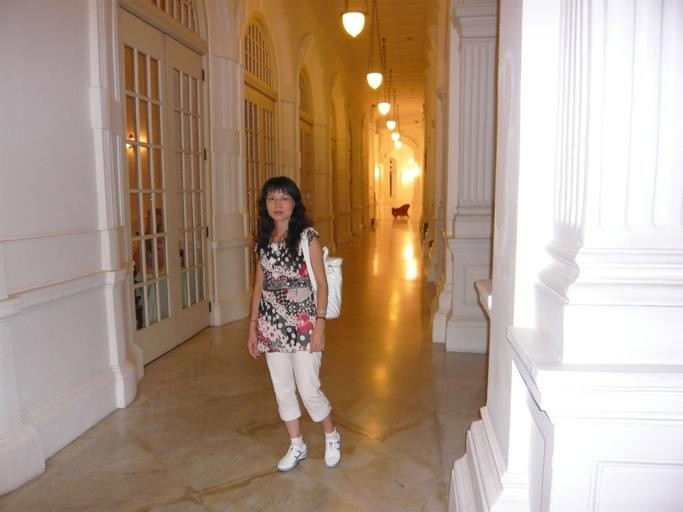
[245,173,341,472]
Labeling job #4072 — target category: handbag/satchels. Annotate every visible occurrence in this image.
[300,227,343,320]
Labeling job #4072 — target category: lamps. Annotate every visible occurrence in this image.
[366,0,384,90]
[378,38,391,115]
[342,0,369,38]
[387,69,402,149]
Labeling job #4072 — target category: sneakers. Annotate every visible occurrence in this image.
[276,447,309,471]
[325,432,340,467]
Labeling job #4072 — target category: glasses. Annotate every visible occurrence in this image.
[266,194,292,203]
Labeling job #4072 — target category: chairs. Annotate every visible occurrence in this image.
[392,204,410,218]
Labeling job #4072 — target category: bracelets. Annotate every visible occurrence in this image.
[250,319,257,322]
[315,316,326,320]
